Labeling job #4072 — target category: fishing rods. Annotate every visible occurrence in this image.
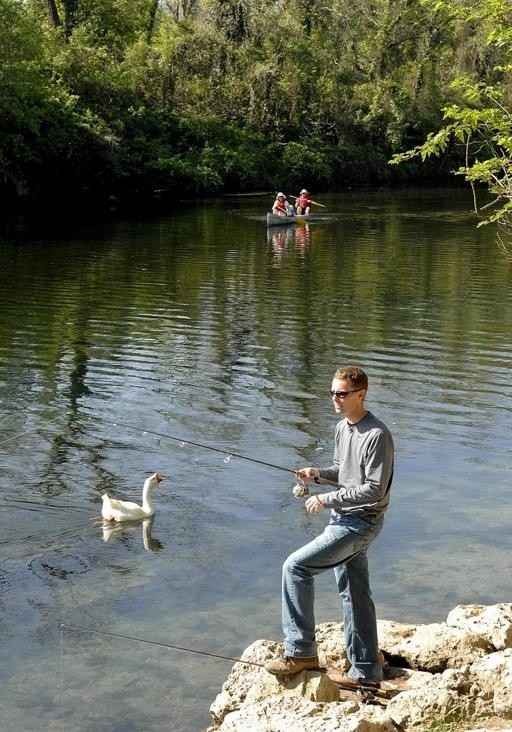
[64,410,341,500]
[63,626,392,703]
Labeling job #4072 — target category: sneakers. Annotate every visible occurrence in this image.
[264,654,319,676]
[328,674,378,694]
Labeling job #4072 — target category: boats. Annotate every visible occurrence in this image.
[267,211,312,226]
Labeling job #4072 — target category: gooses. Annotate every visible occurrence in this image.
[102,519,164,554]
[98,471,165,522]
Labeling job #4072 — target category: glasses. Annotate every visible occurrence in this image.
[330,389,363,398]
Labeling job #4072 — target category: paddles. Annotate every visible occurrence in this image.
[288,193,326,208]
[276,205,307,225]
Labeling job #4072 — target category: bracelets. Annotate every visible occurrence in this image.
[316,494,323,505]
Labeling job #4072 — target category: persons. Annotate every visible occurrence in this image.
[272,228,291,263]
[271,191,290,216]
[262,364,396,689]
[294,188,312,215]
[295,223,311,258]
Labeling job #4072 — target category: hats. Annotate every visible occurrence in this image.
[300,189,307,195]
[276,192,286,200]
